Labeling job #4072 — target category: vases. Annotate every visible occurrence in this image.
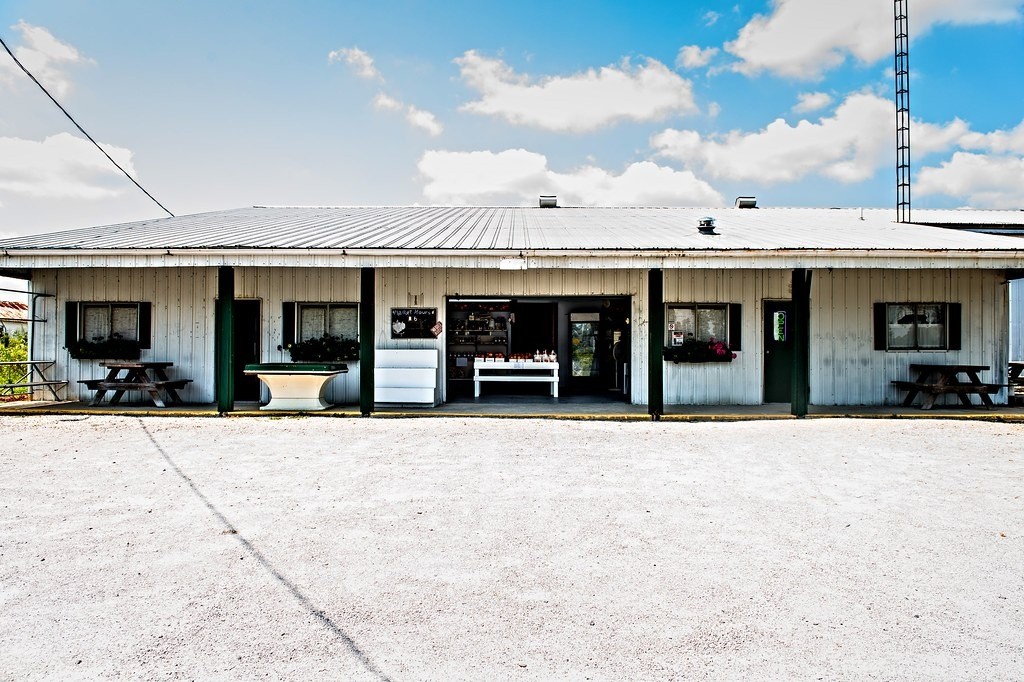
[72,353,140,359]
[299,352,359,361]
[664,353,732,362]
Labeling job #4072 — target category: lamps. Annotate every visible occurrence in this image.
[735,197,757,208]
[539,195,557,208]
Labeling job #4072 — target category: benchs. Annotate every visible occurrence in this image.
[77,378,193,391]
[890,381,1014,394]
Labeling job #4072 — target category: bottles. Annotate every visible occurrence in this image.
[448,336,506,359]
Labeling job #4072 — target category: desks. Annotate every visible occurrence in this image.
[244,363,349,411]
[474,362,559,397]
[902,365,998,411]
[88,362,182,408]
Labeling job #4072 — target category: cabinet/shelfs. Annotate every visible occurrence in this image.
[448,321,511,380]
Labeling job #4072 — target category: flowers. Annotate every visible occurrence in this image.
[662,337,736,364]
[62,332,142,362]
[277,332,360,363]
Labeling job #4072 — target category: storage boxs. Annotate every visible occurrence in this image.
[474,357,504,362]
[509,358,533,362]
